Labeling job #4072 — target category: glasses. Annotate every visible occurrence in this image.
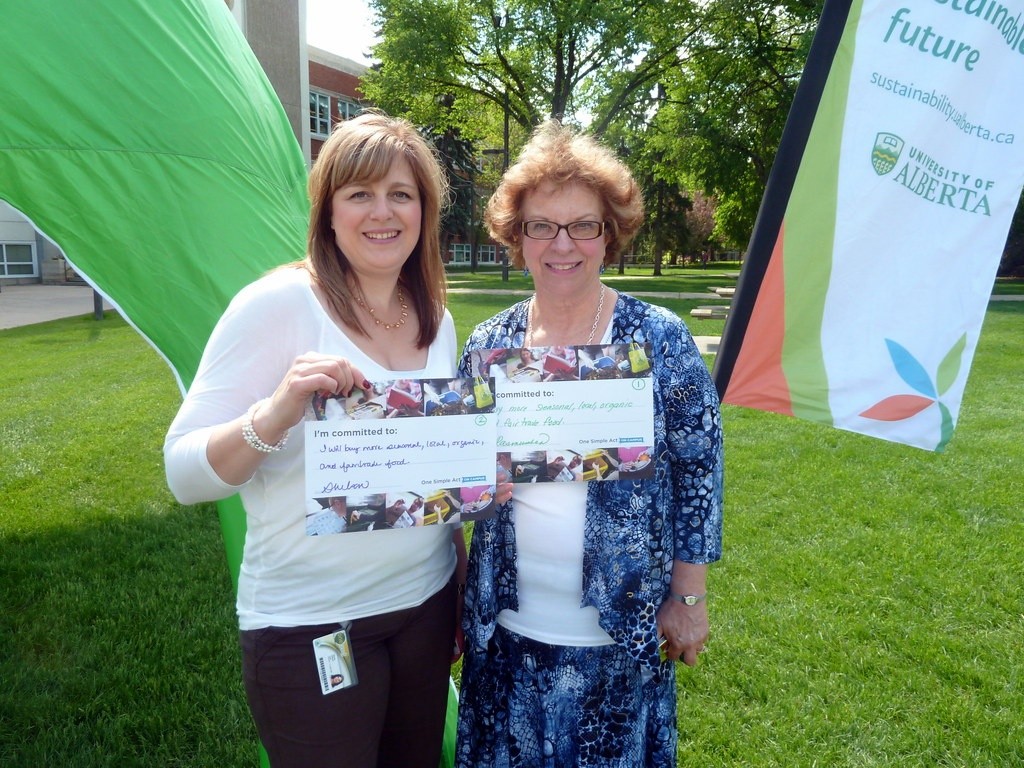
[521,219,605,240]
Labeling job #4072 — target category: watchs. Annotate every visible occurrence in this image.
[670,591,708,606]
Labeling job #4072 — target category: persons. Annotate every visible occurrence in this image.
[454,122,723,768]
[386,498,423,528]
[593,451,619,480]
[547,455,583,482]
[434,491,460,524]
[516,348,538,369]
[357,383,383,405]
[163,114,468,768]
[497,452,511,483]
[331,674,344,688]
[328,497,346,520]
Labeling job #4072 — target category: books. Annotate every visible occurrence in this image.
[387,388,422,412]
[543,355,577,378]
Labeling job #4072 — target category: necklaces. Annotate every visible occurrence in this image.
[528,286,606,347]
[354,283,408,329]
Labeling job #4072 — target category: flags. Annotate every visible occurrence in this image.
[0,0,460,768]
[721,0,1024,455]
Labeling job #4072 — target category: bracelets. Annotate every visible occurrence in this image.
[457,584,465,595]
[243,398,289,453]
